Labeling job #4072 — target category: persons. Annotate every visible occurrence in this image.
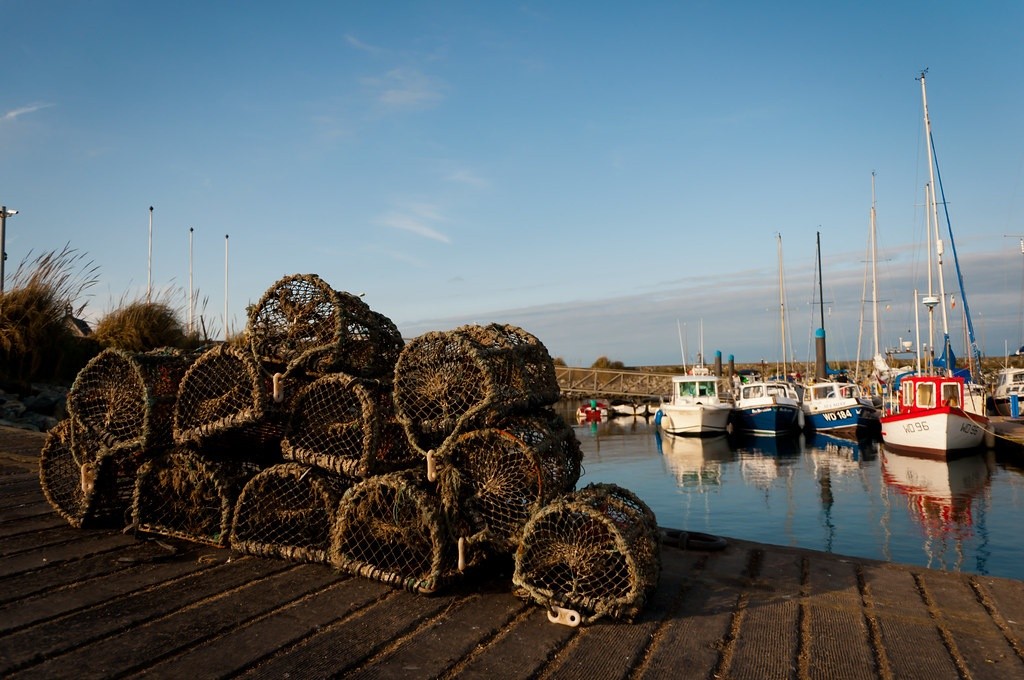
[732,373,740,393]
[837,371,847,383]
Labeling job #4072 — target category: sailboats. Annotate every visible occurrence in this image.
[778,67,1024,457]
[882,444,991,575]
[804,432,878,553]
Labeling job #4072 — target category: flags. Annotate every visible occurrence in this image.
[951,294,955,309]
[1010,346,1024,356]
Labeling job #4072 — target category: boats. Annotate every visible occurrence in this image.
[610,399,647,415]
[576,416,608,432]
[662,430,732,530]
[729,359,801,434]
[727,428,801,509]
[643,398,660,414]
[659,318,733,436]
[576,400,608,416]
[610,413,647,426]
